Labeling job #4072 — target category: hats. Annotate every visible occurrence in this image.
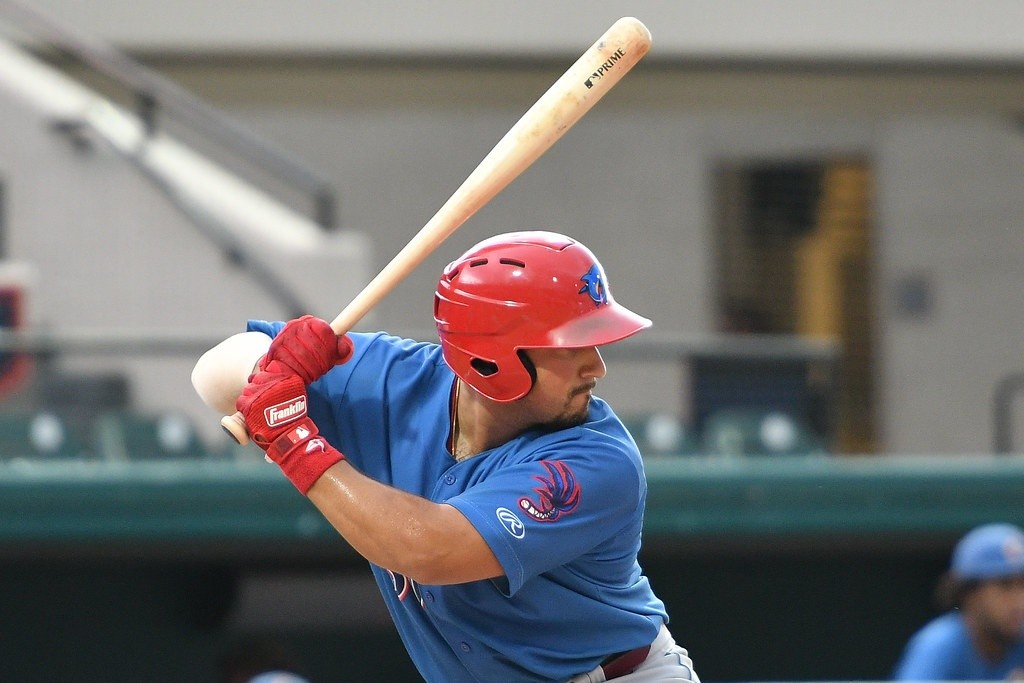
[951,523,1024,581]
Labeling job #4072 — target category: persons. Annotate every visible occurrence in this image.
[192,229,701,683]
[685,297,832,448]
[892,525,1024,683]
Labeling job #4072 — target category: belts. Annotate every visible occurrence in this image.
[600,644,651,681]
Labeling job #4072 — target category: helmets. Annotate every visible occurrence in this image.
[434,229,653,402]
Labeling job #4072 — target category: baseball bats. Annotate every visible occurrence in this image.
[219,15,655,446]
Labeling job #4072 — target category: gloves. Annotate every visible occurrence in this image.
[236,360,345,497]
[258,314,354,386]
